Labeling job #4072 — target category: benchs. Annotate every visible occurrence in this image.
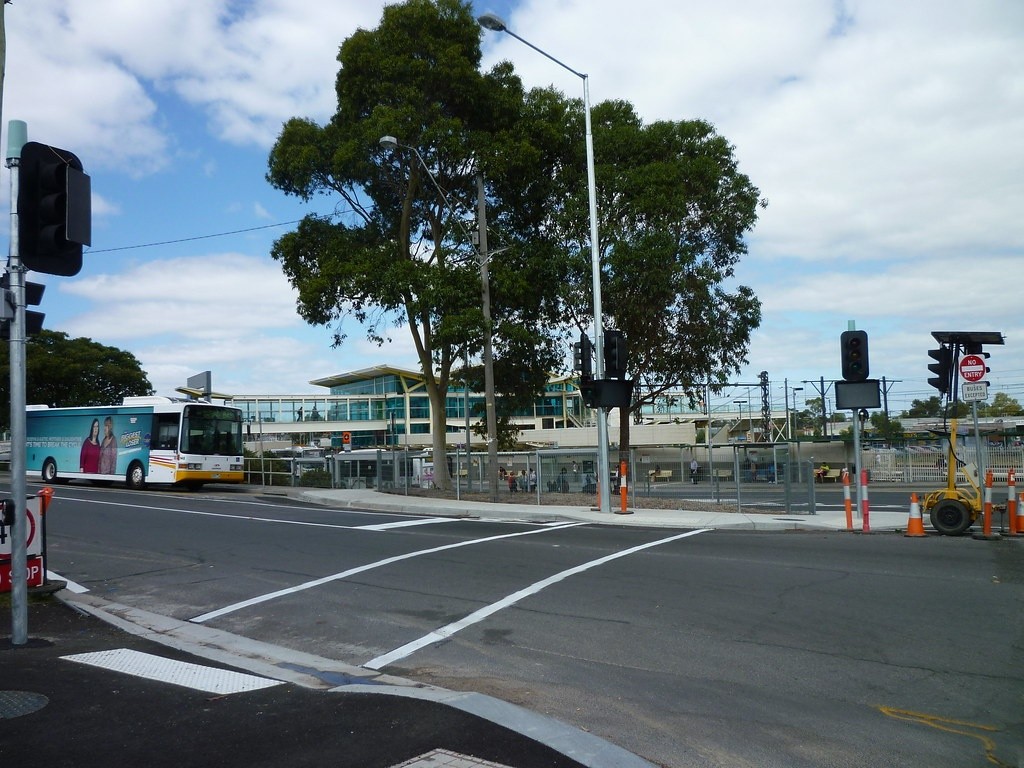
[453,469,468,478]
[517,471,535,479]
[645,469,673,482]
[813,469,840,483]
[498,471,512,479]
[707,468,732,481]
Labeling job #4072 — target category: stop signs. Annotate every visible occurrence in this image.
[960,355,985,381]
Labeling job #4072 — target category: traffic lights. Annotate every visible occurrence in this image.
[0,272,47,342]
[574,332,590,376]
[840,330,869,380]
[927,346,949,393]
[16,141,82,277]
[970,342,990,387]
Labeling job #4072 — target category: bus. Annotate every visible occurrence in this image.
[23,396,252,492]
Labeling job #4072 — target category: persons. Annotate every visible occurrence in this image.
[500,467,507,480]
[690,458,698,484]
[529,470,537,494]
[313,406,318,415]
[80,418,100,474]
[768,462,775,484]
[652,465,660,481]
[99,416,116,475]
[518,470,528,492]
[816,462,830,484]
[573,461,578,482]
[297,407,302,421]
[744,457,751,482]
[509,472,517,492]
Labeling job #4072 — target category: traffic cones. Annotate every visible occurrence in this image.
[1016,492,1024,534]
[904,492,929,538]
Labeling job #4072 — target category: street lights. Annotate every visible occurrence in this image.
[744,387,753,433]
[733,401,747,437]
[791,388,803,440]
[477,12,613,514]
[379,135,499,498]
